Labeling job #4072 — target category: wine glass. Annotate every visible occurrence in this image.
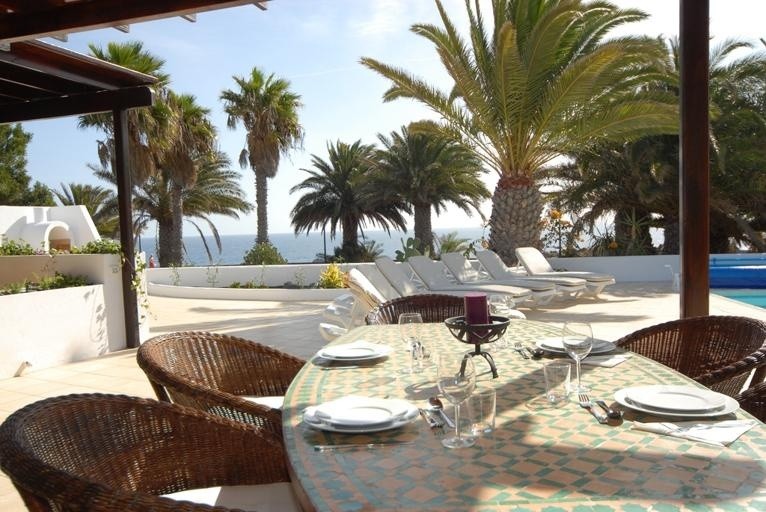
[398,312,425,374]
[489,294,512,350]
[562,320,593,393]
[436,351,477,450]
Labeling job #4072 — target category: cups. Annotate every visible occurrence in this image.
[465,386,497,440]
[543,360,571,403]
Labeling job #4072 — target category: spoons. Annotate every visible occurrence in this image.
[596,400,621,420]
[425,396,457,429]
[526,345,544,357]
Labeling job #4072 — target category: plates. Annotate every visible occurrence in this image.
[535,337,617,354]
[614,385,740,420]
[303,396,419,433]
[318,342,391,362]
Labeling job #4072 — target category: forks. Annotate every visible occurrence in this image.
[419,408,445,429]
[578,394,607,424]
[514,341,530,360]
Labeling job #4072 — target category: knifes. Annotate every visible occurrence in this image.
[314,440,416,451]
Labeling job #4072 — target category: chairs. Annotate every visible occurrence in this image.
[317,246,615,342]
